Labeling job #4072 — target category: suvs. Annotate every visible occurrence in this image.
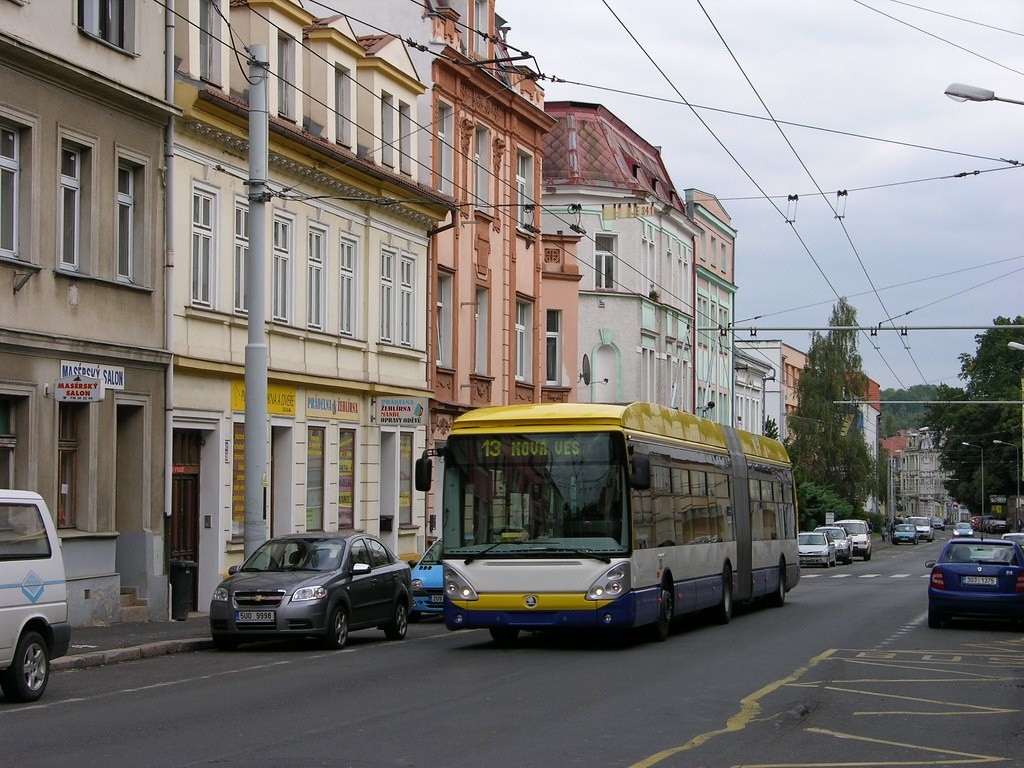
[799,530,836,567]
[808,525,853,565]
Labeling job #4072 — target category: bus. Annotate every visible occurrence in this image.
[415,402,800,642]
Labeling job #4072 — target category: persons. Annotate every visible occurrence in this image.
[881,514,903,545]
[867,518,873,543]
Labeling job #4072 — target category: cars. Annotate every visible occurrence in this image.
[832,520,872,561]
[892,524,919,545]
[908,517,935,542]
[210,532,412,649]
[925,539,1024,628]
[1001,533,1024,552]
[932,517,945,531]
[966,516,1010,534]
[408,534,499,623]
[950,523,976,538]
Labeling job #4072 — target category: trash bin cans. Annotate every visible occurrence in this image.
[170,559,198,620]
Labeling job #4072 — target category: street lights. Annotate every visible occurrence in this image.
[890,450,901,522]
[962,442,984,516]
[993,440,1020,519]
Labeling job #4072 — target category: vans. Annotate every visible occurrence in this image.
[0,489,71,701]
